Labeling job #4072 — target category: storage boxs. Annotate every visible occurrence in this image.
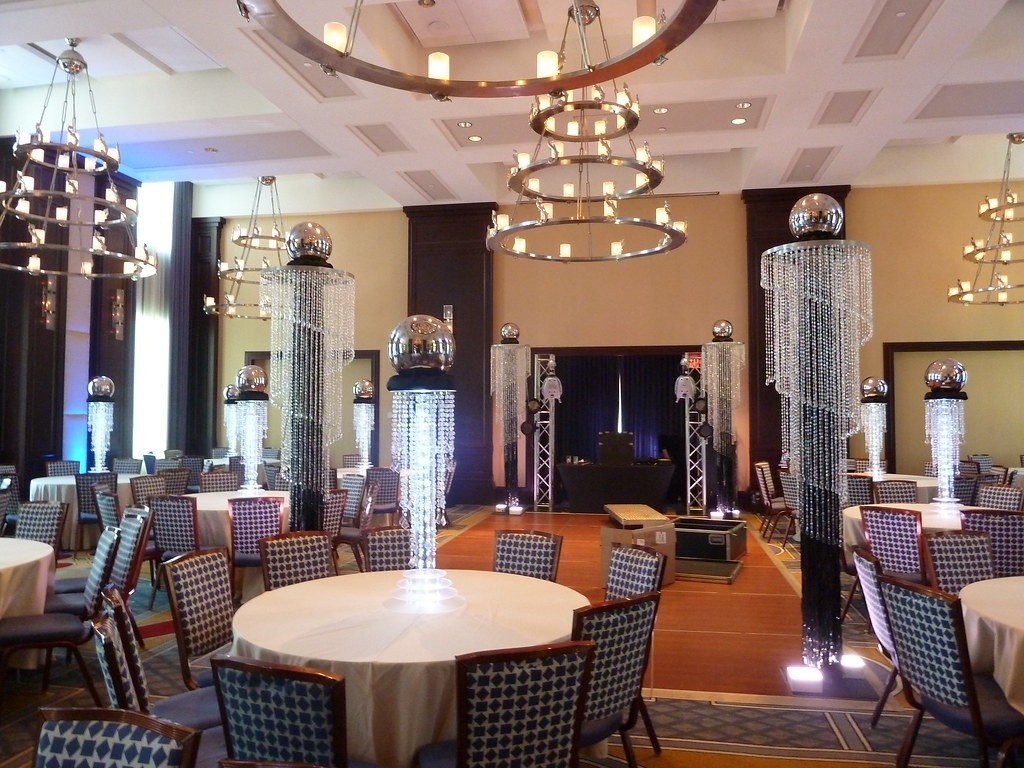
[666,516,748,561]
[600,524,677,589]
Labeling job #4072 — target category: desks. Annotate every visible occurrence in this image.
[0,537,55,671]
[956,574,1023,715]
[842,503,963,570]
[333,468,366,489]
[990,467,1024,492]
[29,474,141,552]
[230,569,596,768]
[556,463,675,515]
[862,472,941,505]
[204,458,281,485]
[180,491,290,560]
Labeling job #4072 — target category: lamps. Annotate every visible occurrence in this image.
[486,0,688,262]
[0,35,158,284]
[945,132,1024,306]
[236,0,717,102]
[203,174,296,322]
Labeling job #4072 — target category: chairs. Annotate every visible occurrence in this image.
[1,448,670,768]
[754,454,1024,768]
